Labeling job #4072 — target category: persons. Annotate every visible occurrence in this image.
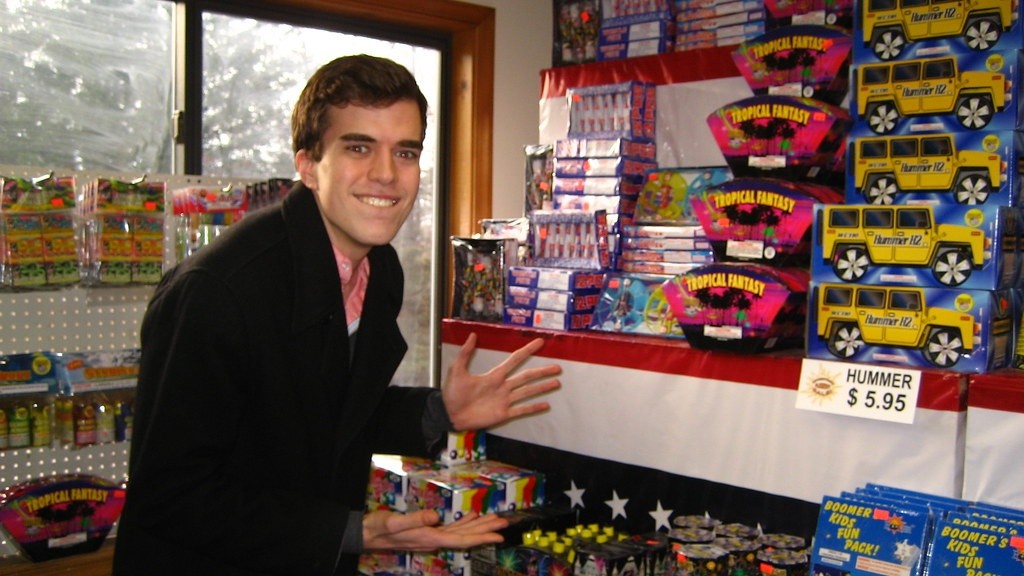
[109,52,562,575]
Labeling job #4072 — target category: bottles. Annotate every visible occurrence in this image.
[519,522,630,576]
[0,395,133,448]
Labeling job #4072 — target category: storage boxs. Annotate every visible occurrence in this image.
[0,473,127,562]
[448,0,1024,372]
[358,430,578,576]
[809,483,1024,576]
